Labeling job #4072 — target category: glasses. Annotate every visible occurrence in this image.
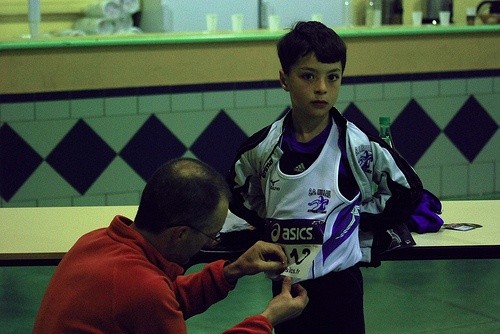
[176,224,224,247]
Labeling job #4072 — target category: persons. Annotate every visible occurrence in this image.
[31,158,310,334]
[226,21,423,334]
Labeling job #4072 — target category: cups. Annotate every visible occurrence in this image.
[411,12,422,25]
[466,8,475,24]
[269,16,279,31]
[439,11,450,24]
[207,15,217,31]
[232,15,243,31]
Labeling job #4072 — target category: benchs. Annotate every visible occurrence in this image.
[0,199,500,268]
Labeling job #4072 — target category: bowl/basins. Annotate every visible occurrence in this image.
[480,14,499,23]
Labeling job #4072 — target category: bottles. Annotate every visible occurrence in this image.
[367,0,381,28]
[378,116,395,150]
